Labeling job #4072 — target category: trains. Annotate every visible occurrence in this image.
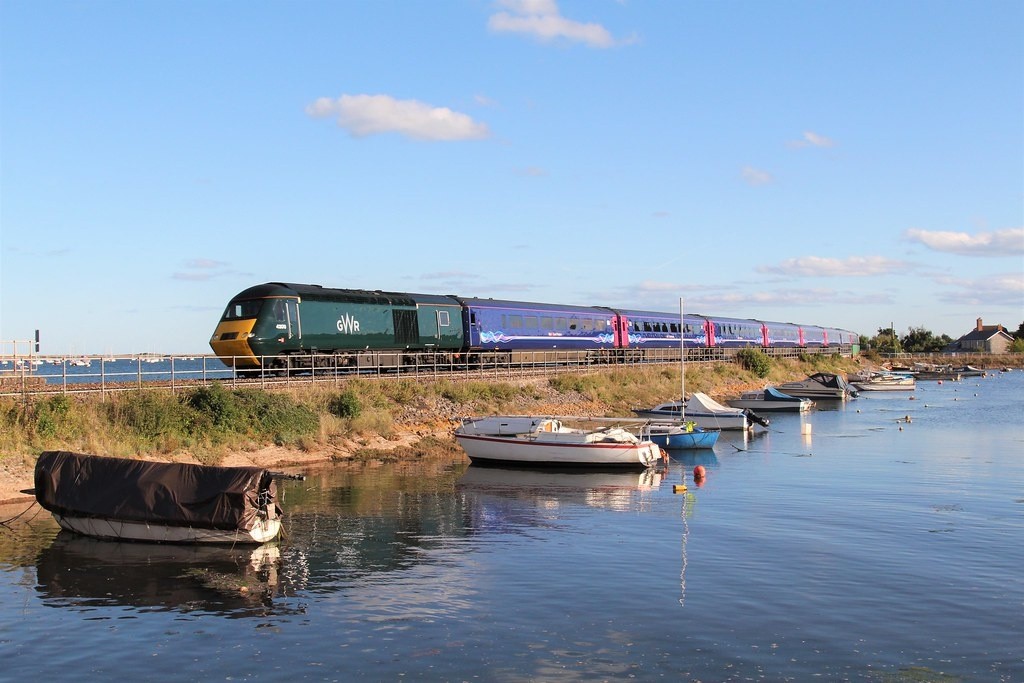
[209,282,860,378]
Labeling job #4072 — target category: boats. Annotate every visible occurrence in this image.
[630,391,770,432]
[33,451,283,544]
[726,388,817,413]
[448,412,686,469]
[846,361,987,391]
[776,372,860,400]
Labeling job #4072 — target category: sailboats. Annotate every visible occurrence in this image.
[633,298,722,452]
